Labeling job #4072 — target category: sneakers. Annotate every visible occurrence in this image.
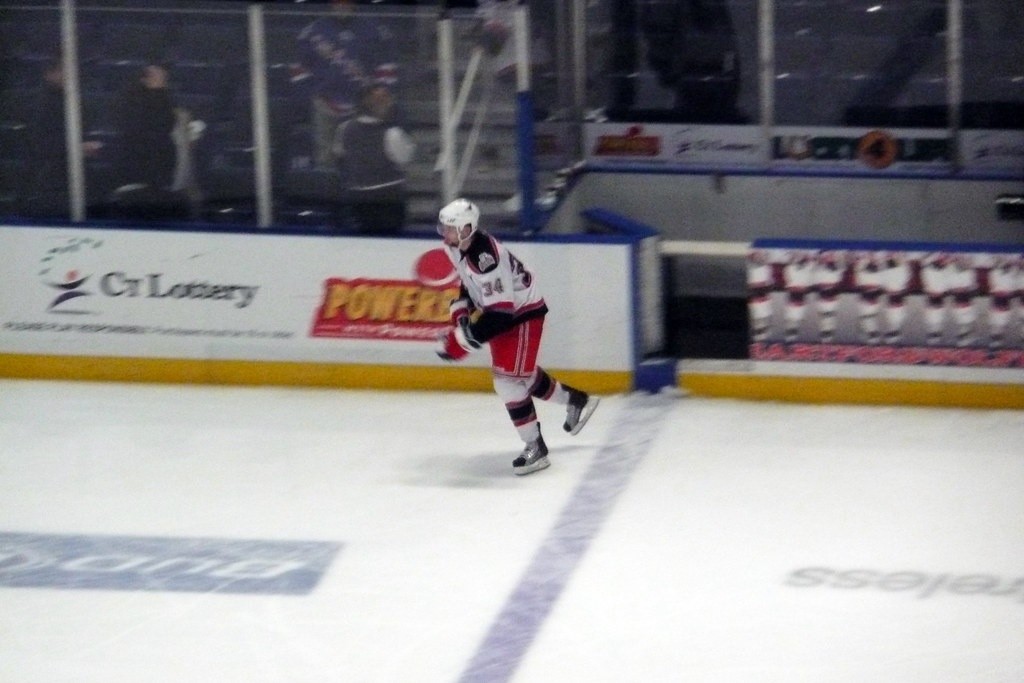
[513,421,551,475]
[560,385,600,436]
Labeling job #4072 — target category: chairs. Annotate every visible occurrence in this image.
[0,0,429,233]
[586,0,1024,130]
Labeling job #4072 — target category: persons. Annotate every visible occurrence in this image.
[469,1,607,123]
[0,0,415,236]
[435,199,589,468]
[630,22,681,122]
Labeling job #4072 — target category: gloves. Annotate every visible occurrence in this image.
[435,323,482,362]
[450,298,470,330]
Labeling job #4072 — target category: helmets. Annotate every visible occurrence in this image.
[439,198,480,236]
